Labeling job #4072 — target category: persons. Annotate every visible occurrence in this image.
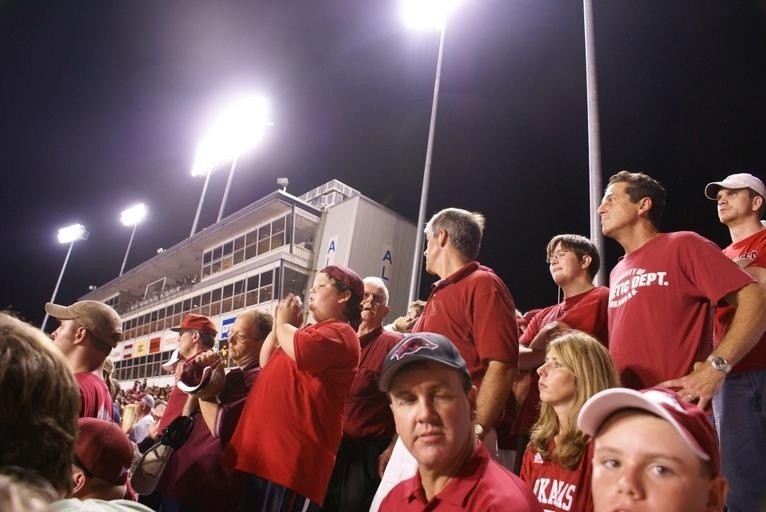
[224,265,366,510]
[383,300,425,335]
[693,173,766,511]
[514,234,609,474]
[596,169,766,424]
[577,386,726,511]
[518,330,620,512]
[307,277,401,510]
[1,300,172,512]
[380,334,542,512]
[370,208,518,512]
[163,275,272,512]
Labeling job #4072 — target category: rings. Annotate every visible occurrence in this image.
[689,395,694,402]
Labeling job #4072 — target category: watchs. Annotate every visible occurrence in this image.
[706,355,731,374]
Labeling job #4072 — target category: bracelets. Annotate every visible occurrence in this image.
[471,424,488,439]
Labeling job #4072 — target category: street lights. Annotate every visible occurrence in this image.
[394,1,462,311]
[38,223,88,333]
[188,90,272,236]
[118,203,146,277]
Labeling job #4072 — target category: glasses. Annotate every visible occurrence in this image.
[227,326,261,344]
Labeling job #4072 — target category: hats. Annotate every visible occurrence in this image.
[378,331,470,394]
[72,348,227,496]
[321,265,364,302]
[576,384,720,479]
[45,300,124,348]
[170,313,218,338]
[704,172,765,201]
